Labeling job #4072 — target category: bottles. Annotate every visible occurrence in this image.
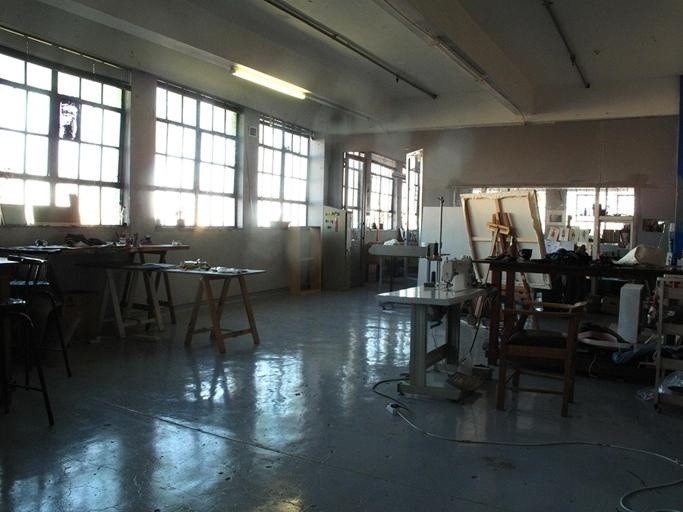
[668,222,675,252]
[584,208,587,216]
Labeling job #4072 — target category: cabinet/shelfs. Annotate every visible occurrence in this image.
[288,225,322,296]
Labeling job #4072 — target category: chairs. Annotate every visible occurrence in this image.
[495,299,586,416]
[0,247,72,424]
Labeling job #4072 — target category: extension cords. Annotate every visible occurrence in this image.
[386,402,401,415]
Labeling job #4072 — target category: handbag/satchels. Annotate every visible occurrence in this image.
[612,244,666,266]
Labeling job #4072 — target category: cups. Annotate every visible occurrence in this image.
[518,249,532,260]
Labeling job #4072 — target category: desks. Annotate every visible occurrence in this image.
[0,242,266,357]
[373,279,487,400]
[472,256,683,363]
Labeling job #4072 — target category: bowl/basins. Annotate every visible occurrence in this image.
[270,221,289,229]
